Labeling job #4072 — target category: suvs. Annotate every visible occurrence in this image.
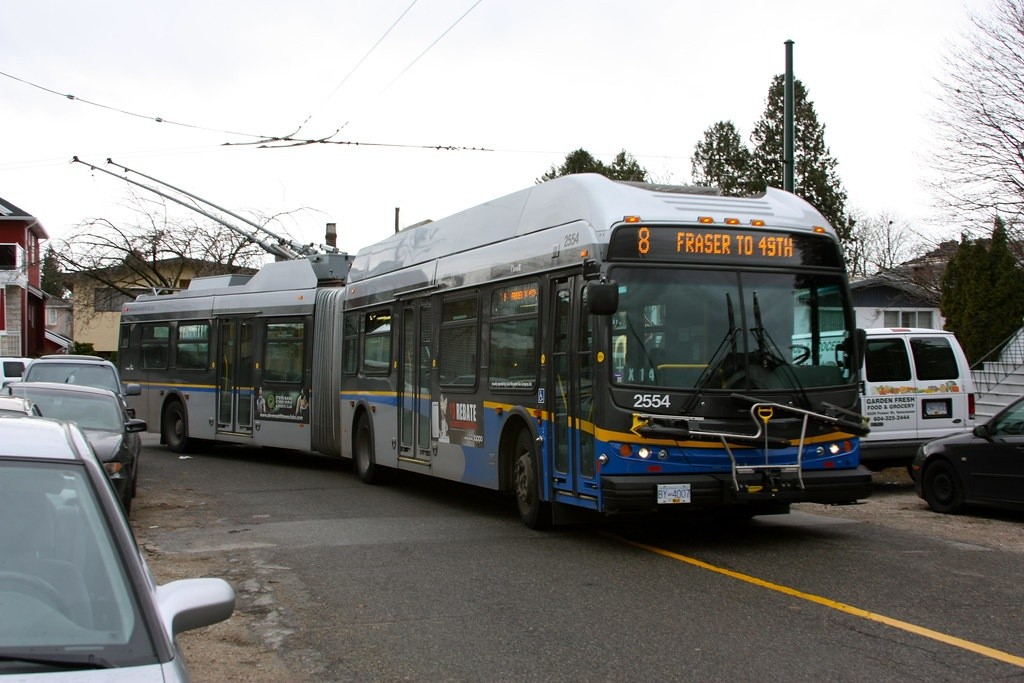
[0,356,38,391]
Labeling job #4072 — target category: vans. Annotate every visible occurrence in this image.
[786,328,977,473]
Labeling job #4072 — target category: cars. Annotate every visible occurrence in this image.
[0,383,147,516]
[911,393,1024,515]
[0,396,44,419]
[22,354,141,416]
[0,415,236,683]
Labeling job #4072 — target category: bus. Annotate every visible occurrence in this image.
[104,171,876,530]
